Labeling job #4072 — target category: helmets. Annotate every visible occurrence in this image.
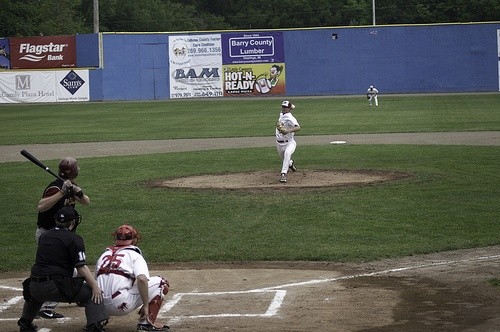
[58,157,78,178]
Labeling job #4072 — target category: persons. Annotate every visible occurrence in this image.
[94,224,169,332]
[367,85,379,107]
[18,205,104,332]
[36,158,90,318]
[276,101,301,182]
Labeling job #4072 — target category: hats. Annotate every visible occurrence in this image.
[113,224,137,245]
[282,101,291,108]
[56,207,76,222]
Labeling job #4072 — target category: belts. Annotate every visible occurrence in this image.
[277,140,288,143]
[30,274,56,282]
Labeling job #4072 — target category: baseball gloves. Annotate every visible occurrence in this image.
[367,96,371,99]
[277,122,287,135]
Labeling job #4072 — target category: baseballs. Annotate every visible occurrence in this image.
[291,105,295,109]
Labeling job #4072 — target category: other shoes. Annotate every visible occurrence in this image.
[39,311,63,321]
[289,160,297,172]
[280,174,288,182]
[87,323,105,332]
[137,322,169,332]
[20,323,37,332]
[83,320,107,329]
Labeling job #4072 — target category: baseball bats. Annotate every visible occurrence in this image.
[20,149,84,199]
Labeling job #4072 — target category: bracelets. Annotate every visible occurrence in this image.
[60,190,66,195]
